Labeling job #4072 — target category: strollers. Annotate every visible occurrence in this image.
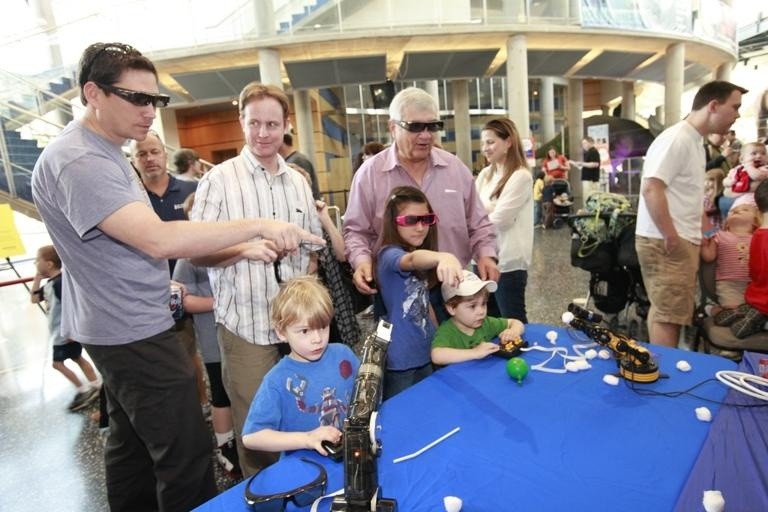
[558,205,701,346]
[548,178,577,230]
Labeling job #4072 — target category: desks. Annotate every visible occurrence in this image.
[187,320,768,511]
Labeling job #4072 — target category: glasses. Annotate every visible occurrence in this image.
[96,82,170,108]
[397,121,445,133]
[396,214,437,227]
[245,458,328,512]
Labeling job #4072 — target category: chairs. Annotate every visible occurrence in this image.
[690,255,767,359]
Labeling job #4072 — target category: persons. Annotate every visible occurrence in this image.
[30,39,361,512]
[631,80,768,351]
[339,87,533,402]
[533,137,600,228]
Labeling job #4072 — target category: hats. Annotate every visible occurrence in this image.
[440,270,500,302]
[283,122,297,138]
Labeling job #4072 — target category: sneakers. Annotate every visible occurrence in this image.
[215,437,242,480]
[730,307,766,340]
[713,303,751,325]
[68,390,98,412]
